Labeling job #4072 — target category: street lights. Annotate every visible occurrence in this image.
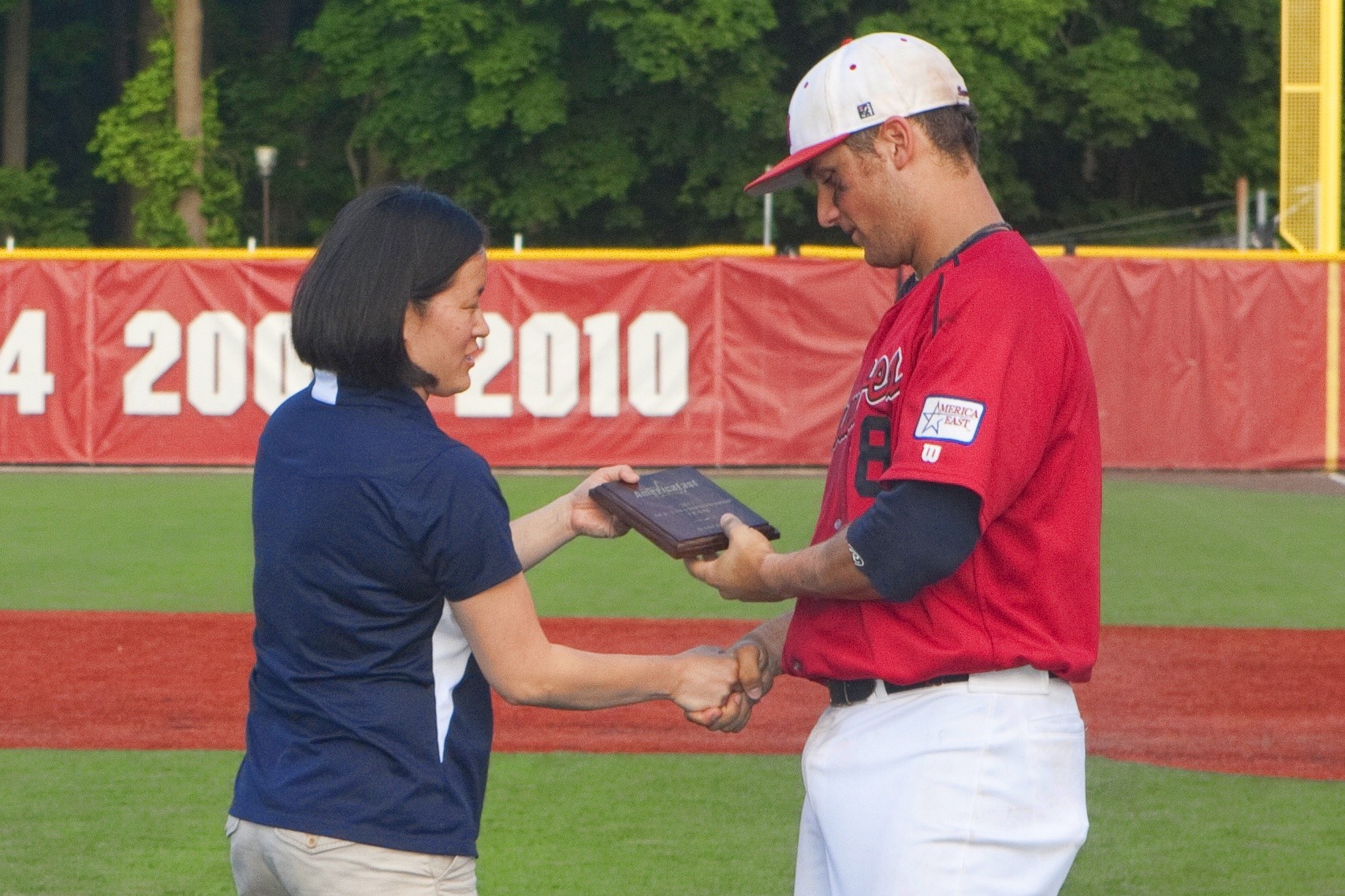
[255,145,279,248]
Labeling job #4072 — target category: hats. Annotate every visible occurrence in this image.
[744,31,969,199]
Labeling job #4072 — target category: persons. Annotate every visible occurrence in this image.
[224,185,745,896]
[684,31,1104,896]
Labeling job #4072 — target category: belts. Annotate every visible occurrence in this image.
[827,667,1057,707]
